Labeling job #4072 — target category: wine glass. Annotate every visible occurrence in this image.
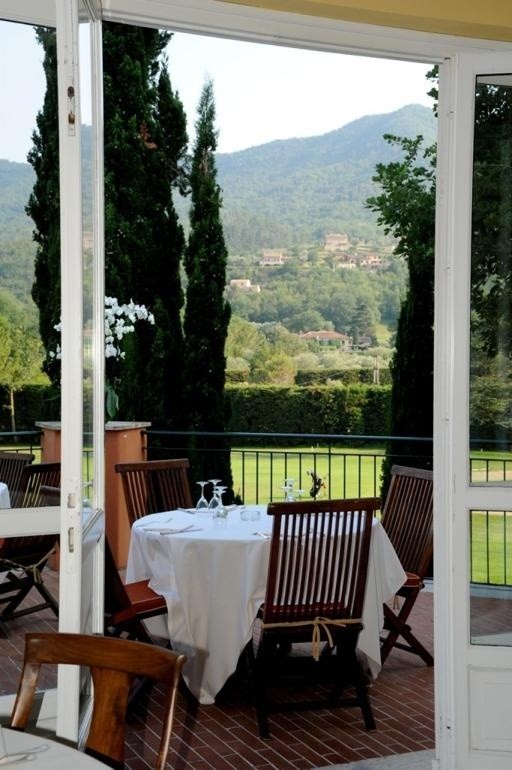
[191,477,307,529]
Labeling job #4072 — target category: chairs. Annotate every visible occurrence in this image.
[0,450,61,638]
[115,459,193,527]
[234,499,381,732]
[0,633,186,769]
[104,531,184,713]
[380,464,433,685]
